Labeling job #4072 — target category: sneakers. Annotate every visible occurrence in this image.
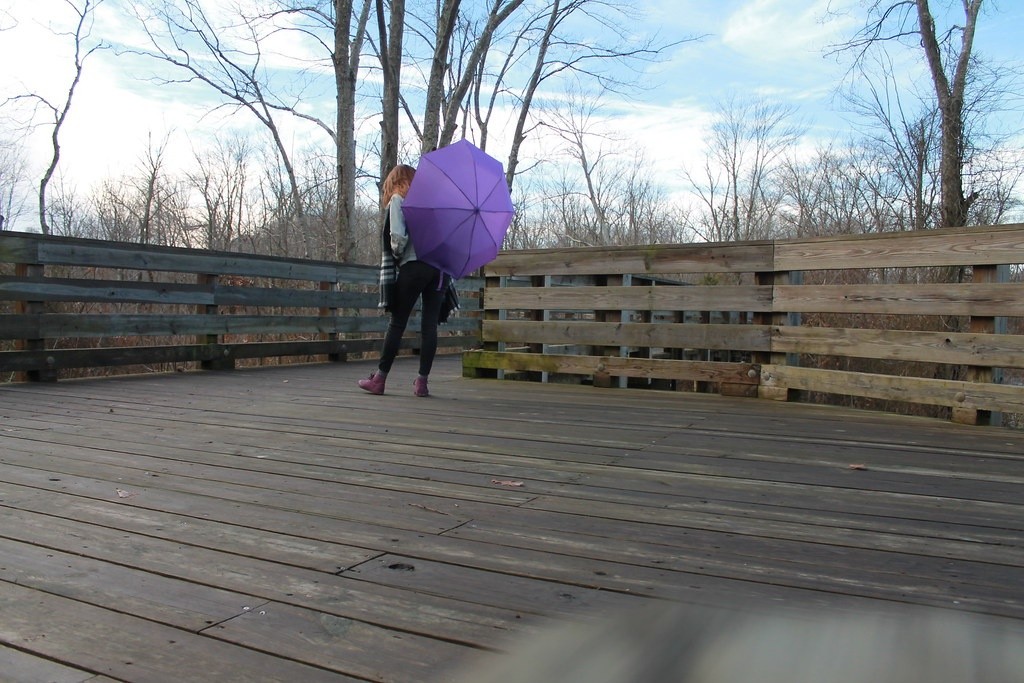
[358,372,386,395]
[413,376,429,396]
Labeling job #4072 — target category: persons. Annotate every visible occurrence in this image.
[358,163,450,398]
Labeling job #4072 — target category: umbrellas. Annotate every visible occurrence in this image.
[400,138,515,278]
